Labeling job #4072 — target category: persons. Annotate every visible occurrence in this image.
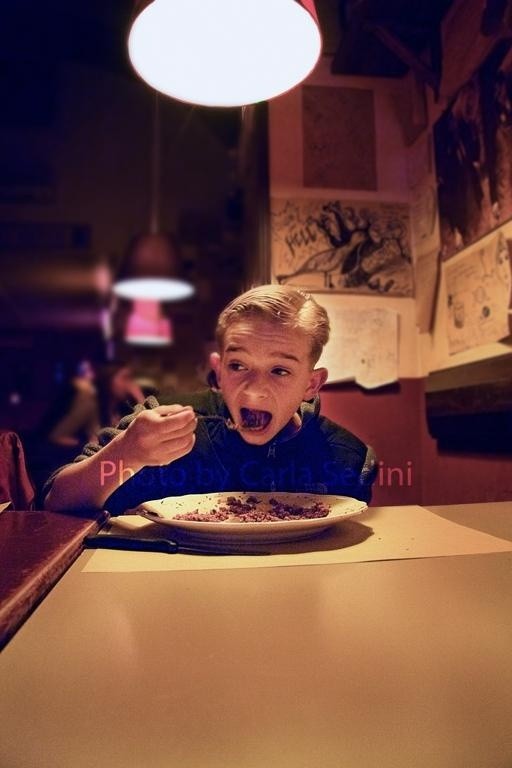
[1,341,216,512]
[25,286,380,524]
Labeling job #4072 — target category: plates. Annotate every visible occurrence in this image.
[138,488,370,547]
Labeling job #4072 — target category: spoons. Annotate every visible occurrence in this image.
[197,414,263,433]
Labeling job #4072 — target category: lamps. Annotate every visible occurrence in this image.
[127,0,324,109]
[110,93,196,348]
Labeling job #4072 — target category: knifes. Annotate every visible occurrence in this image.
[83,536,271,555]
